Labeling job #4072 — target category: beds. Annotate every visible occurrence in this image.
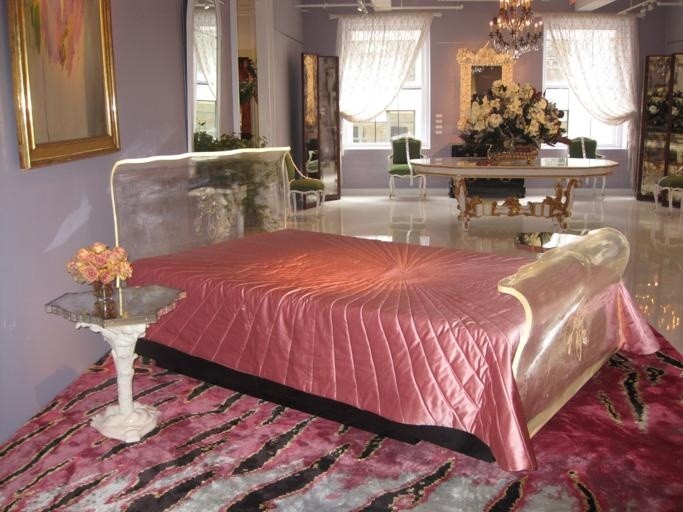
[109,146,633,445]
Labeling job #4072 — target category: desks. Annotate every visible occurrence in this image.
[40,286,191,446]
[409,157,620,233]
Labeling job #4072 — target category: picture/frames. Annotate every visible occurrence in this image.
[456,40,514,129]
[8,2,122,172]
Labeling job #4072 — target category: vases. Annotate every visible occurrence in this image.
[489,142,540,165]
[92,286,118,317]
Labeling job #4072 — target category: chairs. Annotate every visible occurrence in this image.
[387,136,427,199]
[281,151,326,218]
[657,174,682,219]
[565,136,608,199]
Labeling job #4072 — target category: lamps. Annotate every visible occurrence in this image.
[487,0,545,55]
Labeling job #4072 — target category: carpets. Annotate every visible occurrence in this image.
[3,330,683,508]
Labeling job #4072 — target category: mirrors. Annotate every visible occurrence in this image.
[635,54,683,201]
[301,51,342,210]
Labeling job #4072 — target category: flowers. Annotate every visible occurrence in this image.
[459,79,564,149]
[68,240,132,318]
